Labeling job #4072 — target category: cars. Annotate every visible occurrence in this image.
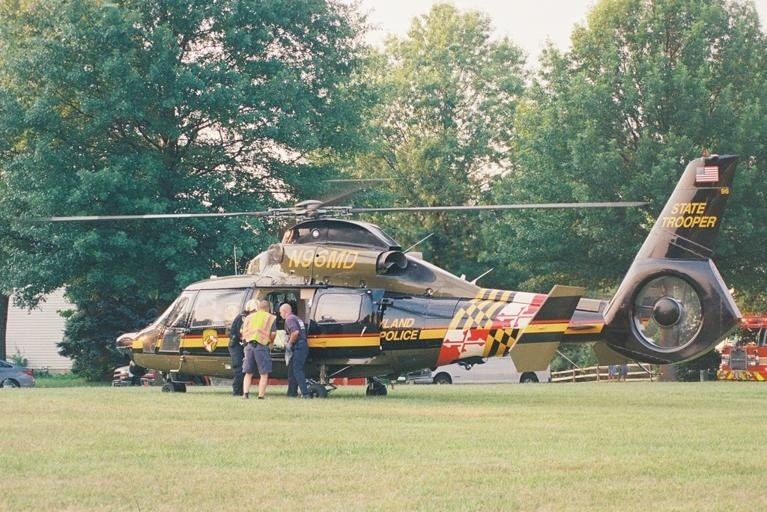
[0,359,36,388]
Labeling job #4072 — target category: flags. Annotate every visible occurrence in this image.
[696,166,719,182]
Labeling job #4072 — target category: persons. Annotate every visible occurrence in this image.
[228,299,277,399]
[608,362,628,383]
[279,303,310,399]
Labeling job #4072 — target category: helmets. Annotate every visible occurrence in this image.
[246,299,260,311]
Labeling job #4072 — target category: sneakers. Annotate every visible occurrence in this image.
[233,392,248,399]
[258,397,265,399]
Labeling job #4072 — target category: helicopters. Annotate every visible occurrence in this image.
[37,153,746,398]
